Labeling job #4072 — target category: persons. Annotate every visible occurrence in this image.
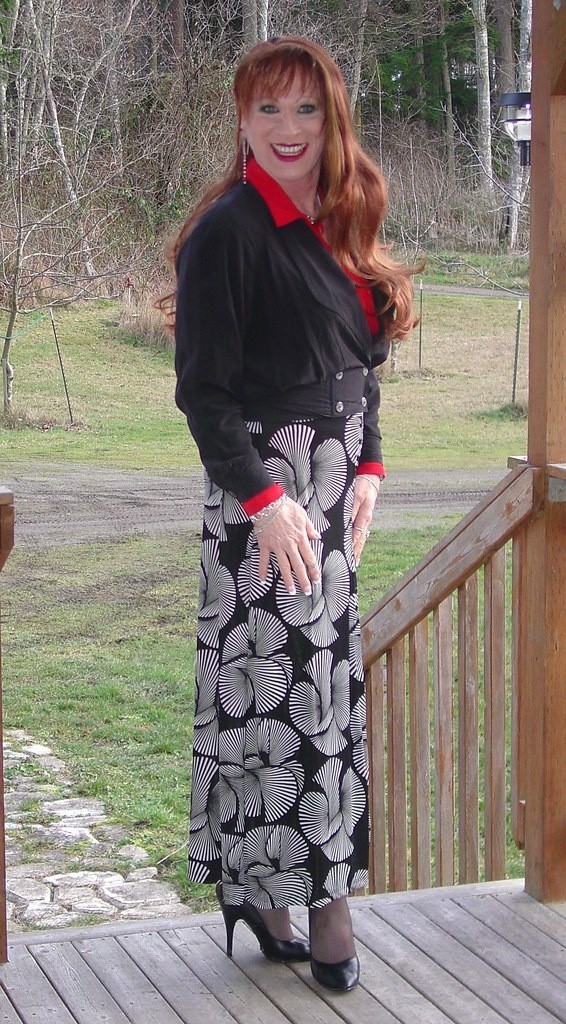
[158,37,428,993]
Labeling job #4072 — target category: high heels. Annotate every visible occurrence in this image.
[309,899,359,992]
[216,880,311,962]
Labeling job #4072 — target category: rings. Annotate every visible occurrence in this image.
[353,526,363,534]
[365,530,370,538]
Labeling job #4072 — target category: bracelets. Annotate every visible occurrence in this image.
[356,475,380,493]
[250,493,289,537]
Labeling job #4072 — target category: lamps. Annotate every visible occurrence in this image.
[498,92,531,166]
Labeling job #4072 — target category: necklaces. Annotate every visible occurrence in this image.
[305,192,324,226]
